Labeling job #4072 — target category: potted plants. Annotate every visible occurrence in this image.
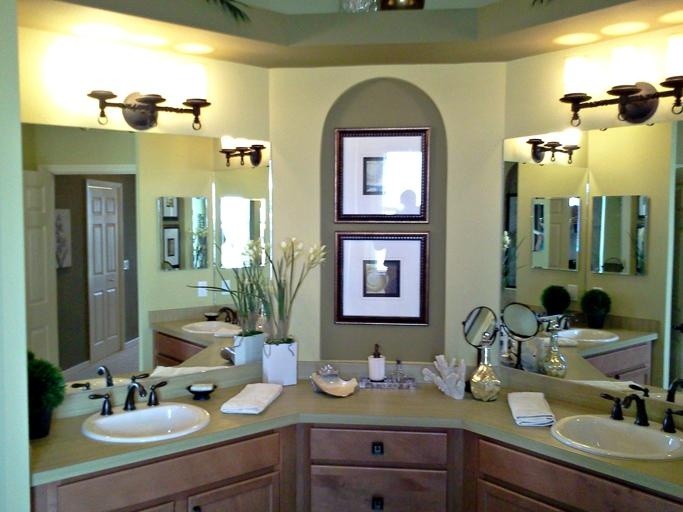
[578,289,617,327]
[26,357,67,440]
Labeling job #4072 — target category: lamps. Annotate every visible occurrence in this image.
[558,72,682,128]
[86,88,209,138]
[526,139,582,168]
[217,134,269,168]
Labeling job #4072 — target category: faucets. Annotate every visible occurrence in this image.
[124,381,146,411]
[557,314,577,330]
[622,392,649,427]
[96,366,112,387]
[219,307,238,325]
[667,378,683,403]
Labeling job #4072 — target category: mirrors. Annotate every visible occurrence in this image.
[497,128,683,408]
[590,194,649,273]
[157,196,207,270]
[500,300,543,373]
[460,304,497,393]
[529,196,581,274]
[20,120,275,382]
[217,193,268,269]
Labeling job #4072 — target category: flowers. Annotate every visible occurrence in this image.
[186,240,265,340]
[500,227,530,288]
[232,235,329,346]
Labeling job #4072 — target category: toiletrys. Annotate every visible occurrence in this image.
[389,357,410,384]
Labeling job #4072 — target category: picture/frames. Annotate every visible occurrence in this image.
[534,203,545,233]
[162,224,180,268]
[333,126,435,228]
[160,197,181,222]
[333,231,433,329]
[533,233,544,253]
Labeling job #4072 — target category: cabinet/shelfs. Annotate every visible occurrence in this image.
[149,329,206,366]
[306,426,449,510]
[51,431,280,512]
[477,438,655,510]
[584,343,653,386]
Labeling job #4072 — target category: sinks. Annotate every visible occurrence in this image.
[80,401,209,444]
[550,326,620,344]
[181,321,245,338]
[550,411,683,462]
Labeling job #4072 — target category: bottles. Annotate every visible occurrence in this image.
[542,332,567,378]
[470,331,501,402]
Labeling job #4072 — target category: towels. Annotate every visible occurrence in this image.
[214,327,242,338]
[570,377,643,394]
[505,389,555,428]
[149,360,231,378]
[217,382,284,415]
[540,334,580,350]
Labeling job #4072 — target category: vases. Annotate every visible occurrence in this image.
[261,339,299,386]
[231,333,265,365]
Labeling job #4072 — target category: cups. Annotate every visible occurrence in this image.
[367,355,387,381]
